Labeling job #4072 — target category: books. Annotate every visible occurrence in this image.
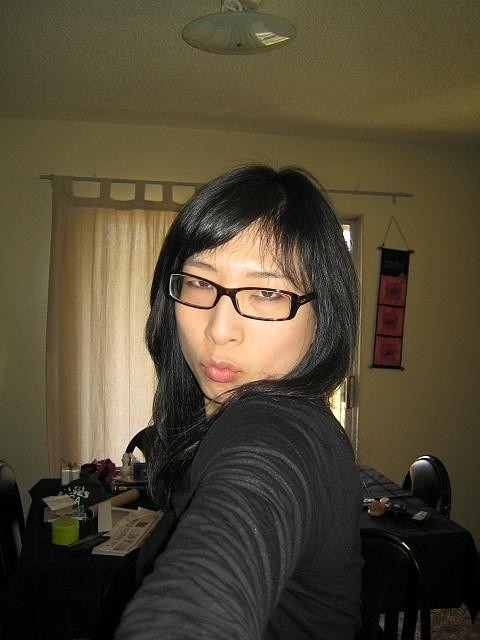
[43,494,79,521]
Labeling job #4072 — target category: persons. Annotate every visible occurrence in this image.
[112,161,367,640]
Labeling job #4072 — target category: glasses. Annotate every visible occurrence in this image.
[161,268,317,325]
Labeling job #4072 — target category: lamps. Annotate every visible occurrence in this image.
[177,0,300,59]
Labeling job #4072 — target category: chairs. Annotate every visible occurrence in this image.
[0,455,38,640]
[350,525,435,640]
[398,446,453,520]
[119,423,164,476]
